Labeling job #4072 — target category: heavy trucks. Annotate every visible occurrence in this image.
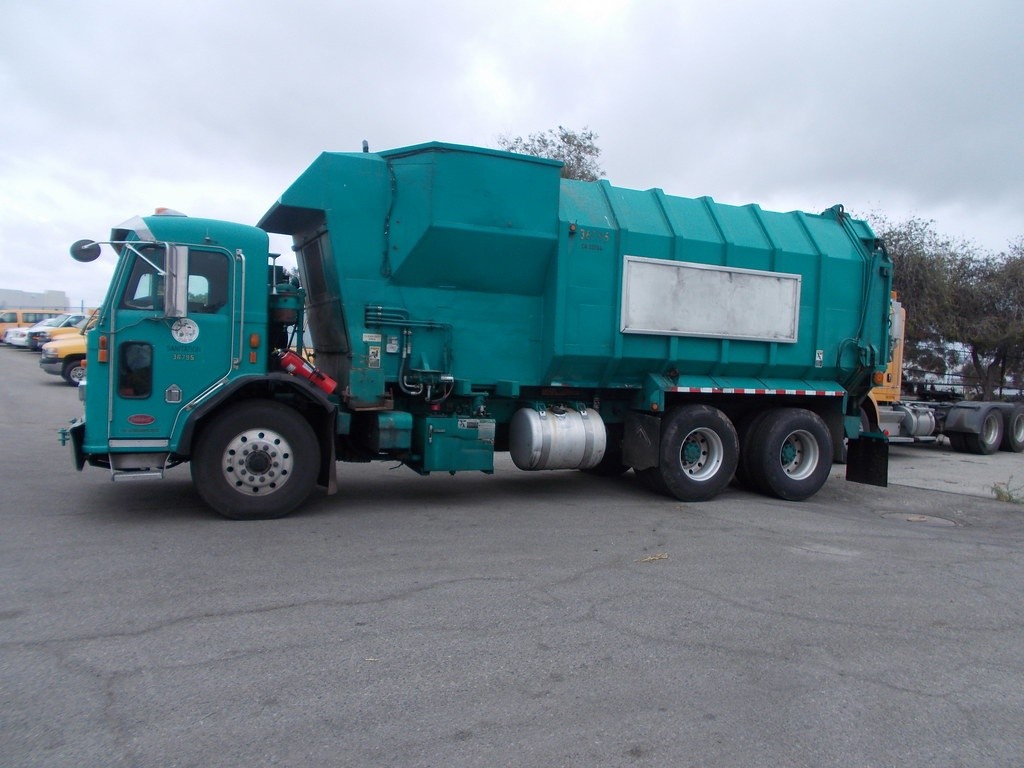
[835,291,1024,463]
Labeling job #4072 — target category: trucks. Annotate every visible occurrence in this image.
[58,139,893,521]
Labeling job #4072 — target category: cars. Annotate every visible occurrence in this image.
[4,326,30,347]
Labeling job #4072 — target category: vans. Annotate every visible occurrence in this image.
[0,309,65,342]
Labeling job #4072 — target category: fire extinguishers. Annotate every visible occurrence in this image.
[272,347,338,395]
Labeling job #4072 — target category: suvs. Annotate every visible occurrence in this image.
[29,310,100,387]
